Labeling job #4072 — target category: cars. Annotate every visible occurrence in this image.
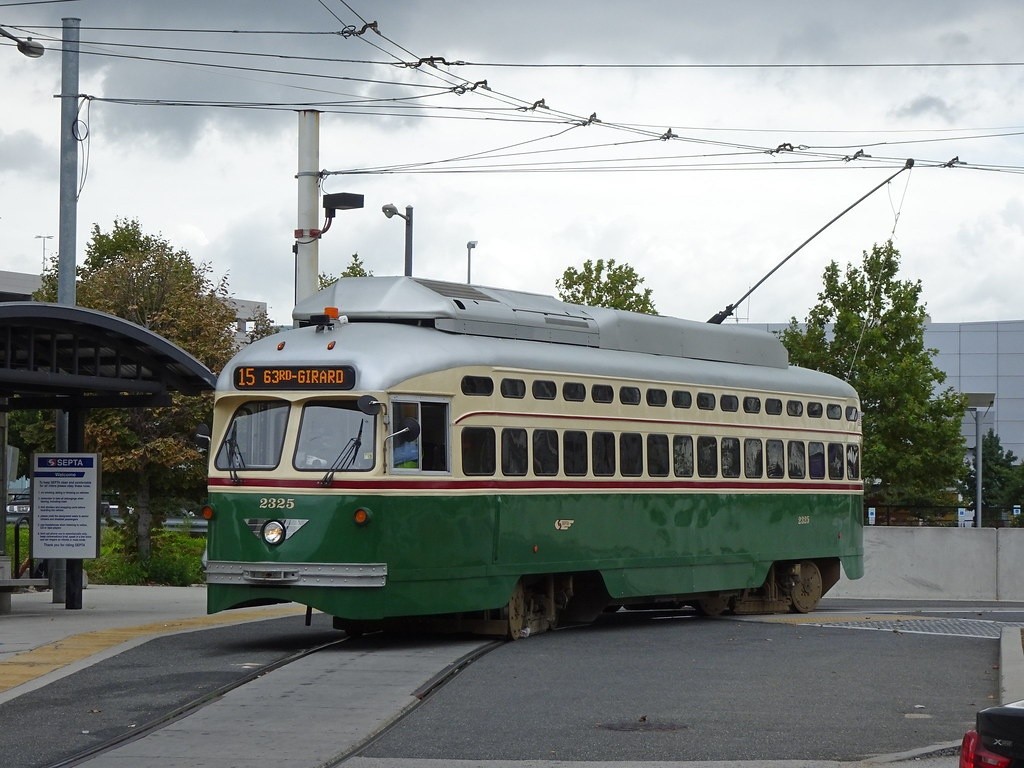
[959,697,1024,768]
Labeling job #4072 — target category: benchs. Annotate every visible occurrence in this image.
[0,577,51,615]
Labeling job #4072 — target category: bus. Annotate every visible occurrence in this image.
[196,276,865,643]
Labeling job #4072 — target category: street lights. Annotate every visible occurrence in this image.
[466,240,479,284]
[380,203,414,277]
